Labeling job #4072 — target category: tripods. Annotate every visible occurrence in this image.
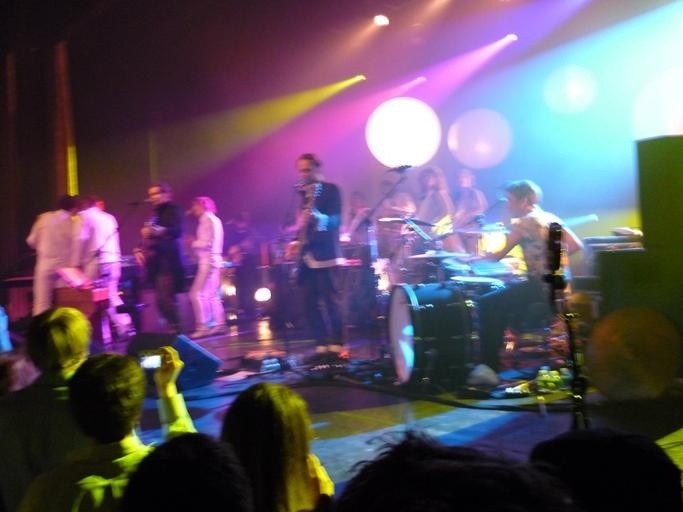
[246,287,309,378]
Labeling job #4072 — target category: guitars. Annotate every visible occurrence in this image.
[132,219,158,287]
[290,182,321,280]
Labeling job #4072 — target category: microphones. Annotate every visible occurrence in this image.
[484,197,509,215]
[545,223,561,274]
[144,198,156,203]
[386,165,412,174]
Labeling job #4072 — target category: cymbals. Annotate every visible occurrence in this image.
[409,252,470,257]
[378,216,432,232]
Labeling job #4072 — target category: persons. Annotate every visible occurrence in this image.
[1,154,681,510]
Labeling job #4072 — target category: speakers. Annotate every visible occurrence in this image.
[603,250,683,379]
[637,134,683,247]
[127,333,222,392]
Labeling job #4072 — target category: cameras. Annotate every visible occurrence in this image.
[137,350,164,370]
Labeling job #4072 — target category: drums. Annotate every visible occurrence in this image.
[388,283,479,388]
[451,275,504,297]
[463,228,505,262]
